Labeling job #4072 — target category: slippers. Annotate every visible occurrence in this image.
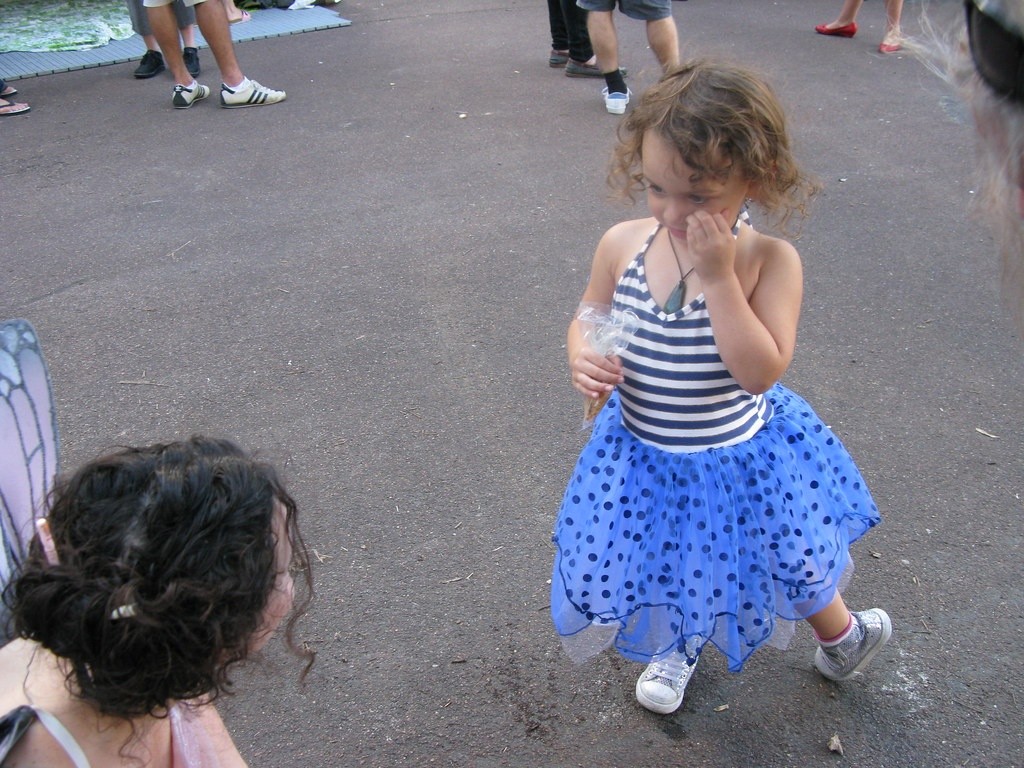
[0,86,18,96]
[228,10,251,25]
[0,100,30,115]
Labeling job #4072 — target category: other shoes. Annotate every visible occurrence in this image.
[549,51,569,68]
[564,58,627,78]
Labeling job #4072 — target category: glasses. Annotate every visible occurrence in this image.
[965,0,1023,100]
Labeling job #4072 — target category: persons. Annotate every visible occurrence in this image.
[223,0,252,25]
[551,60,892,715]
[0,77,31,115]
[547,0,627,78]
[815,0,905,53]
[143,0,286,108]
[577,0,680,115]
[0,434,317,768]
[126,0,200,78]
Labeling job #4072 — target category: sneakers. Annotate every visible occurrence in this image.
[171,81,209,109]
[183,47,200,75]
[134,50,165,76]
[602,85,630,114]
[814,608,892,681]
[635,634,700,715]
[219,80,287,108]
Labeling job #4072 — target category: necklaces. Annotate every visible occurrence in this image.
[663,229,694,314]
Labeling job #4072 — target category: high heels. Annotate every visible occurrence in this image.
[815,23,857,38]
[879,42,901,52]
